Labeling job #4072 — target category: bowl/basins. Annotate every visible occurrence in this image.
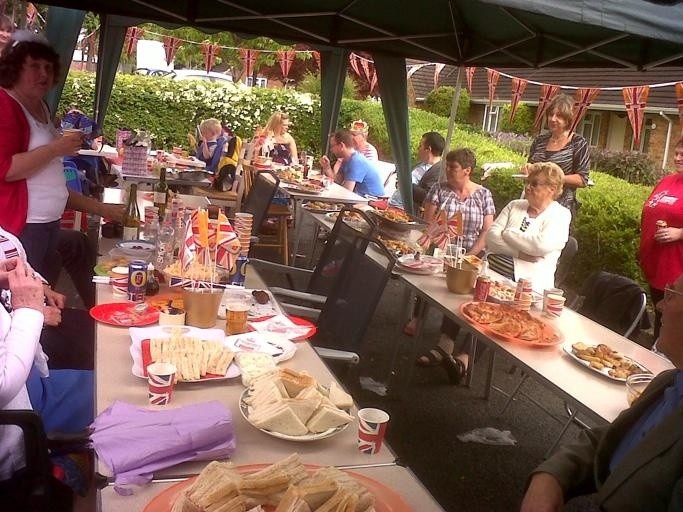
[115,240,155,257]
[141,462,411,512]
[625,373,655,406]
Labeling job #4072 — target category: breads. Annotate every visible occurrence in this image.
[571,342,641,379]
[169,452,377,512]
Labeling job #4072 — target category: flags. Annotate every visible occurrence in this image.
[360,59,370,84]
[349,52,361,79]
[88,33,97,55]
[200,43,222,74]
[25,1,37,27]
[314,51,321,69]
[179,207,217,292]
[239,49,260,78]
[449,213,463,268]
[486,68,500,105]
[82,39,86,49]
[426,210,453,267]
[370,64,378,94]
[417,232,431,251]
[434,63,446,88]
[274,51,295,79]
[465,67,476,92]
[569,89,601,132]
[215,207,242,290]
[164,37,183,67]
[125,27,145,58]
[623,87,648,144]
[510,78,528,126]
[533,85,559,131]
[675,82,683,132]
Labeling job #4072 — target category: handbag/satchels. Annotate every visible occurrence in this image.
[272,144,292,166]
[55,113,104,154]
[116,129,151,156]
[122,145,147,176]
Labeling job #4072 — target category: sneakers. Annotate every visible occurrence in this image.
[313,260,343,277]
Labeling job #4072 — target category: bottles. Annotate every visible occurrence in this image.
[230,256,248,286]
[128,261,148,293]
[146,261,159,296]
[303,157,312,181]
[121,183,140,241]
[655,220,667,234]
[474,275,491,303]
[153,167,169,222]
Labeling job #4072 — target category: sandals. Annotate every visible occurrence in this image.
[442,357,467,386]
[418,346,455,367]
[404,319,418,336]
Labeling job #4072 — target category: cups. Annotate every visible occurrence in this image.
[146,363,176,405]
[148,147,189,171]
[357,407,389,454]
[144,206,158,224]
[234,212,253,257]
[62,129,81,153]
[256,156,272,170]
[514,277,532,298]
[225,303,249,334]
[158,307,185,325]
[111,267,128,297]
[299,151,313,170]
[546,293,566,318]
[446,244,466,256]
[543,288,563,312]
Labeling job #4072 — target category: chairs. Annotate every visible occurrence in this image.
[226,169,279,244]
[494,269,647,462]
[190,142,254,213]
[277,233,396,382]
[246,207,376,304]
[412,235,578,355]
[0,409,79,512]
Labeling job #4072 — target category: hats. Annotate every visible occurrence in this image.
[350,119,368,133]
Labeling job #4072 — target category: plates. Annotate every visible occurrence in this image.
[249,315,316,342]
[394,254,443,275]
[487,290,531,313]
[373,240,422,260]
[531,300,540,305]
[366,206,432,231]
[270,167,326,194]
[93,263,109,276]
[168,265,225,281]
[487,292,513,303]
[89,303,159,326]
[301,203,353,213]
[561,341,656,382]
[460,301,565,349]
[342,210,371,235]
[224,330,297,368]
[397,253,443,269]
[145,294,183,310]
[324,210,340,222]
[127,325,240,382]
[238,383,352,442]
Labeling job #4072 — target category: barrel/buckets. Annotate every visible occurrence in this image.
[182,285,224,328]
[446,262,477,294]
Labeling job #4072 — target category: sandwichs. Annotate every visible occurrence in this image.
[242,366,356,436]
[149,336,236,382]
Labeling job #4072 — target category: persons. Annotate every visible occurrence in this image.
[0,18,12,50]
[0,224,94,368]
[415,162,571,384]
[389,132,446,213]
[316,131,387,200]
[320,120,379,179]
[403,148,495,336]
[191,119,221,163]
[638,140,683,357]
[0,258,94,482]
[524,276,682,507]
[252,113,299,164]
[0,41,127,293]
[519,95,591,228]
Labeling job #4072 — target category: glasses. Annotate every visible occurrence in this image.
[330,144,341,148]
[664,283,683,301]
[280,114,289,119]
[525,178,551,187]
